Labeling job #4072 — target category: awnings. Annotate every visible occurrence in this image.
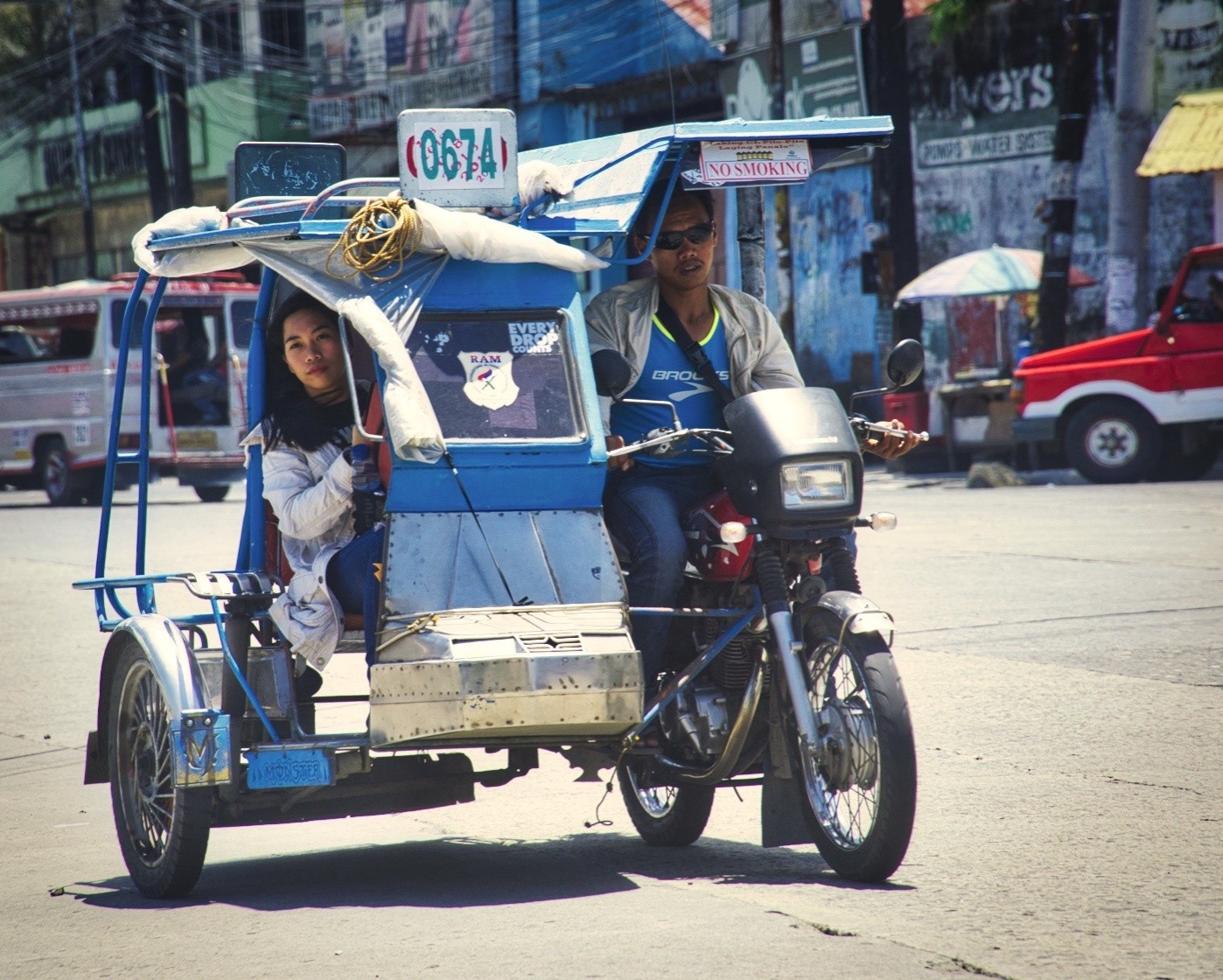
[1136,84,1223,177]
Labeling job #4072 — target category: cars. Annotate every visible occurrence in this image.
[1006,243,1222,487]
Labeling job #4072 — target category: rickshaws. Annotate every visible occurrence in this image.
[82,105,920,903]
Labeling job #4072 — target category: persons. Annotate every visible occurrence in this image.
[169,335,228,403]
[160,307,209,409]
[582,163,922,770]
[1146,285,1185,325]
[239,287,385,733]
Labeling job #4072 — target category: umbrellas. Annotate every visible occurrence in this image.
[891,241,1099,367]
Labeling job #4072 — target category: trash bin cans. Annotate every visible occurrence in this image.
[943,374,1033,472]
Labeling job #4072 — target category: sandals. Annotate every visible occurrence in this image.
[626,719,664,756]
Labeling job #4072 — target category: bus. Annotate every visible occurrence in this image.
[1,268,261,510]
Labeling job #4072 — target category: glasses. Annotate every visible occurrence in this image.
[640,223,713,250]
